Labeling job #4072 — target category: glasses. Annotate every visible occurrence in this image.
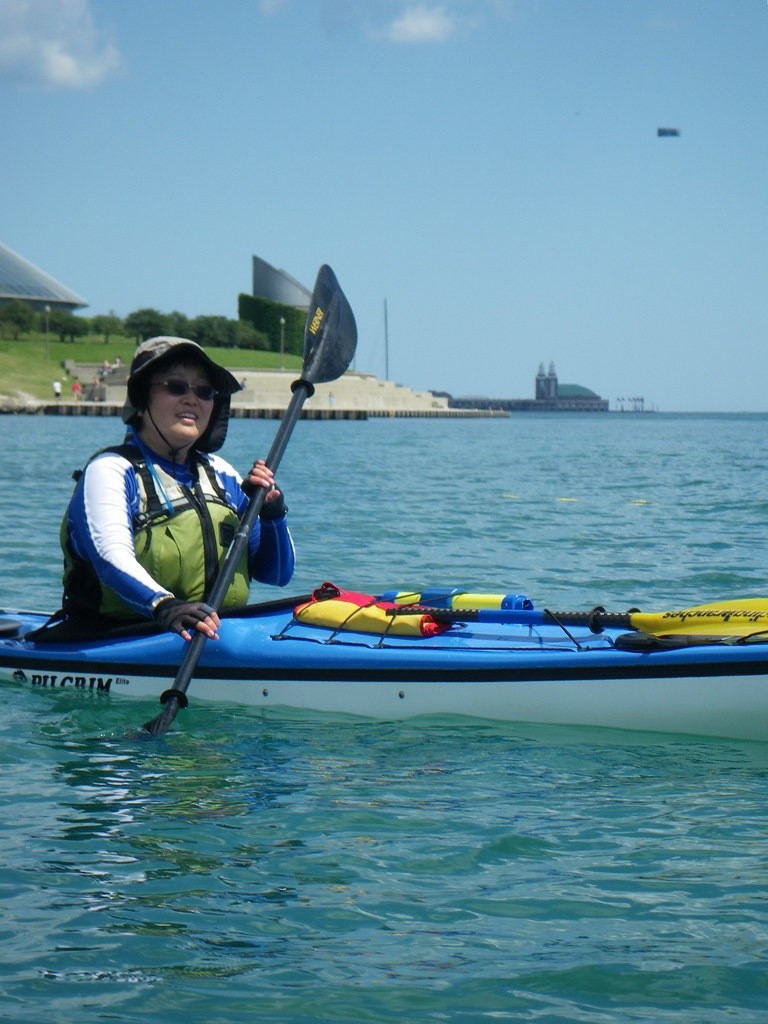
[149,379,220,401]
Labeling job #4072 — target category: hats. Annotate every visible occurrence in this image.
[122,336,243,453]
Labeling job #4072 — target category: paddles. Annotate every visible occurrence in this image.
[122,263,363,731]
[385,596,768,643]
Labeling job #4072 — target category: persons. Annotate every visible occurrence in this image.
[60,336,296,641]
[104,360,110,368]
[72,380,81,401]
[53,379,62,401]
[116,356,121,365]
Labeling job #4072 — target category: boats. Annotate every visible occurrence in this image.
[0,582,768,735]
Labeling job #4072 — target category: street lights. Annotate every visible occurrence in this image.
[280,318,285,370]
[45,305,51,360]
